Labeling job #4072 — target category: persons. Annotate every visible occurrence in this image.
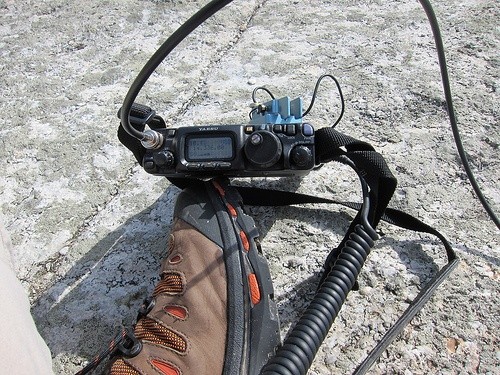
[73,174,284,375]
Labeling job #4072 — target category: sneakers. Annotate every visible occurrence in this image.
[90,177,284,375]
